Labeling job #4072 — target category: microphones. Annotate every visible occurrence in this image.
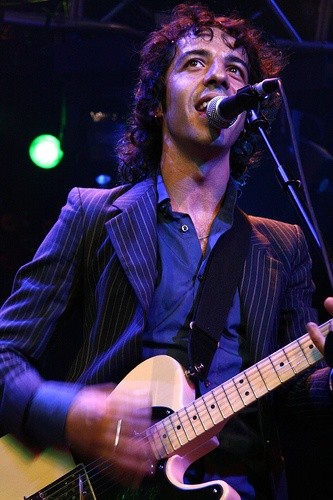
[207,78,279,129]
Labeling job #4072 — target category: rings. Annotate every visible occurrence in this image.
[113,418,123,447]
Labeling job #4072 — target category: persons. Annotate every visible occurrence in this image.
[0,8,333,500]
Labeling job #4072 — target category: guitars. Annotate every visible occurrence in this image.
[0,317,333,500]
